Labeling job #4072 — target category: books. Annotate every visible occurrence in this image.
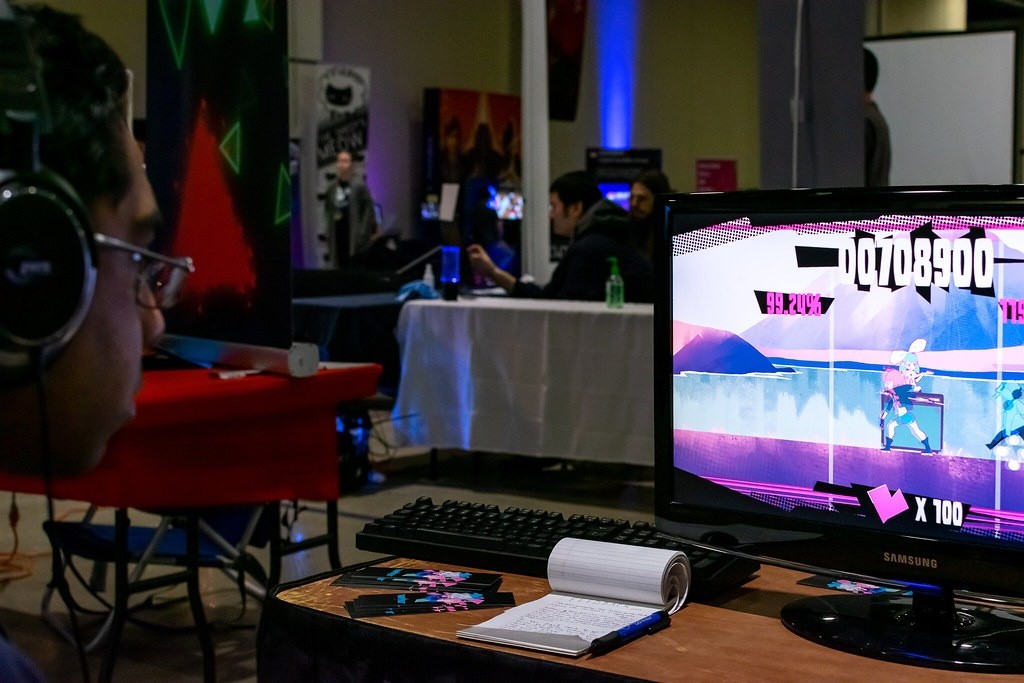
[452,534,693,660]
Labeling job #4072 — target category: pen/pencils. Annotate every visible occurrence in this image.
[587,609,673,659]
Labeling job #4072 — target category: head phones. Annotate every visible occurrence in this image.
[0,18,101,367]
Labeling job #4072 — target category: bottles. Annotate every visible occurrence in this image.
[605,257,624,310]
[423,264,435,288]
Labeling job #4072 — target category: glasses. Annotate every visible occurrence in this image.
[91,226,190,312]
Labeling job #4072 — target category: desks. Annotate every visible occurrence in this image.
[256,556,1024,683]
[290,293,400,396]
[389,292,655,471]
[0,351,383,683]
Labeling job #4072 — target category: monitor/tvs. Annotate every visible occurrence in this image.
[653,185,1024,674]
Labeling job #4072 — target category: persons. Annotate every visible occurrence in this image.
[324,147,379,274]
[0,0,172,683]
[863,46,892,188]
[437,118,505,180]
[459,169,684,414]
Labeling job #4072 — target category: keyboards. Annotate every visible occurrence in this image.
[354,496,760,595]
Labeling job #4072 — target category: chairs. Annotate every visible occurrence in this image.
[39,506,268,652]
